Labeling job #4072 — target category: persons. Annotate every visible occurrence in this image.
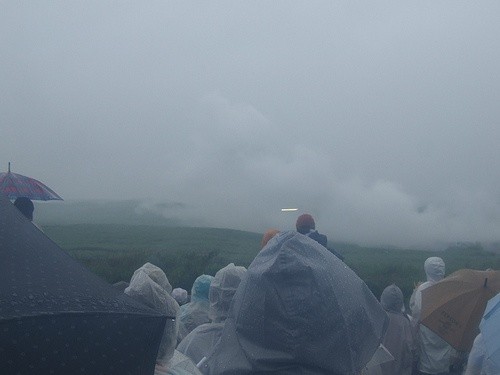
[296,213,327,246]
[112,229,499,374]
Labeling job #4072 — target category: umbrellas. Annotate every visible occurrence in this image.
[420,268,500,353]
[0,194,176,375]
[0,161,66,202]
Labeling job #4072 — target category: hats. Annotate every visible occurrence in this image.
[296,214,315,232]
[171,288,188,305]
[263,229,279,246]
